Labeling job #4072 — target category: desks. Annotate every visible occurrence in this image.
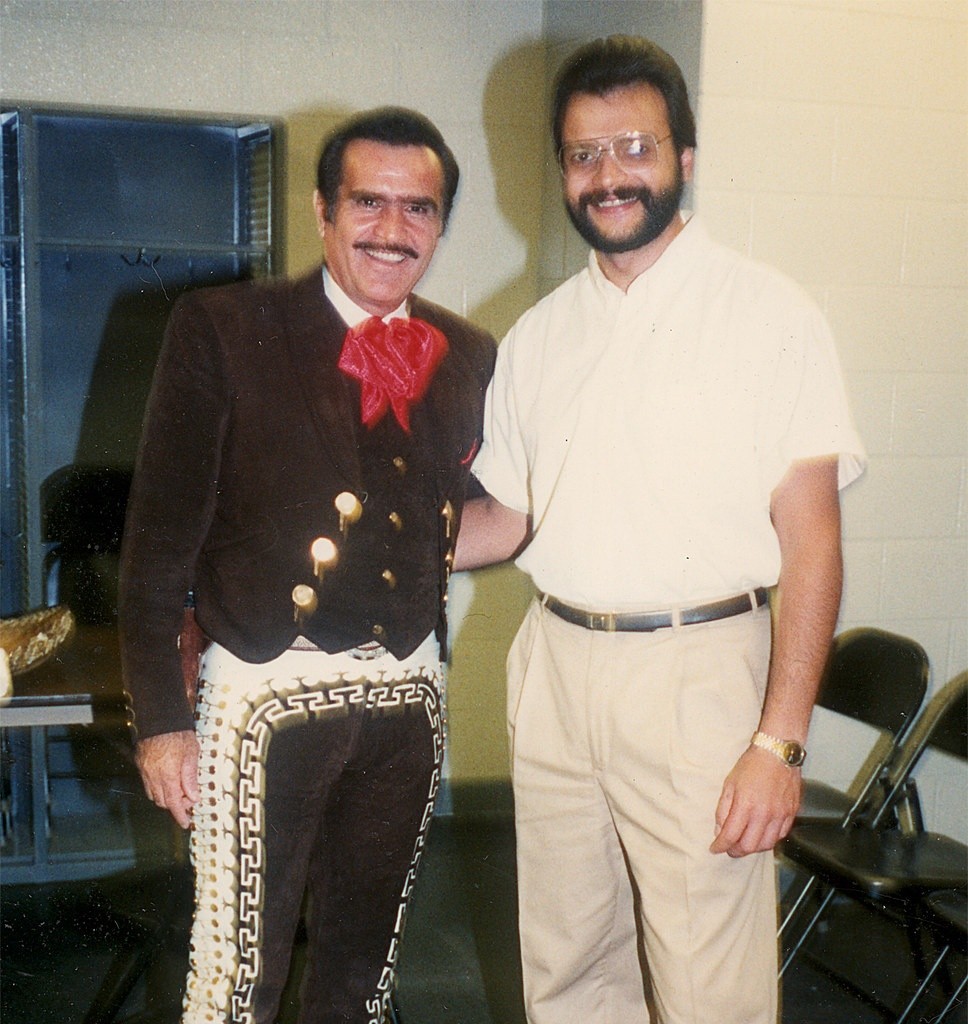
[1,626,126,726]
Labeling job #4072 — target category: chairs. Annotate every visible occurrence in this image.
[776,625,968,1024]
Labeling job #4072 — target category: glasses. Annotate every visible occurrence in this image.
[558,131,674,183]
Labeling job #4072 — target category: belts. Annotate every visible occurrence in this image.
[534,586,767,633]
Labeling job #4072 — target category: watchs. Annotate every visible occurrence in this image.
[751,732,807,767]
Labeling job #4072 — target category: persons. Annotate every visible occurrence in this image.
[449,38,868,1024]
[119,107,498,1024]
